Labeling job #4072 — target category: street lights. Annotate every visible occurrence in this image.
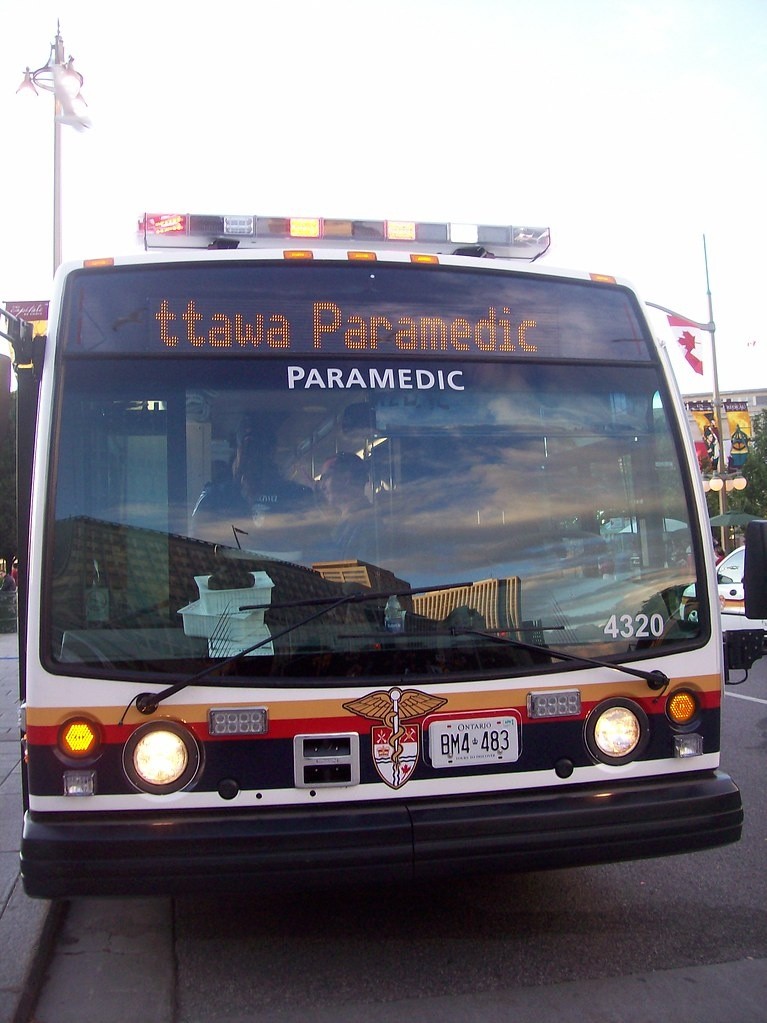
[701,468,746,555]
[14,18,88,273]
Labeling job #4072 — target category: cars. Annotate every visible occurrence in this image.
[678,544,767,634]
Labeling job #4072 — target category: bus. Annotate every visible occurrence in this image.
[56,609,544,678]
[289,389,644,656]
[20,211,766,897]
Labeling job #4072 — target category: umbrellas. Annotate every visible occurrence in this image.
[618,517,687,534]
[707,509,763,551]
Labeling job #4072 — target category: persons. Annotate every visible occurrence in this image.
[183,417,389,590]
